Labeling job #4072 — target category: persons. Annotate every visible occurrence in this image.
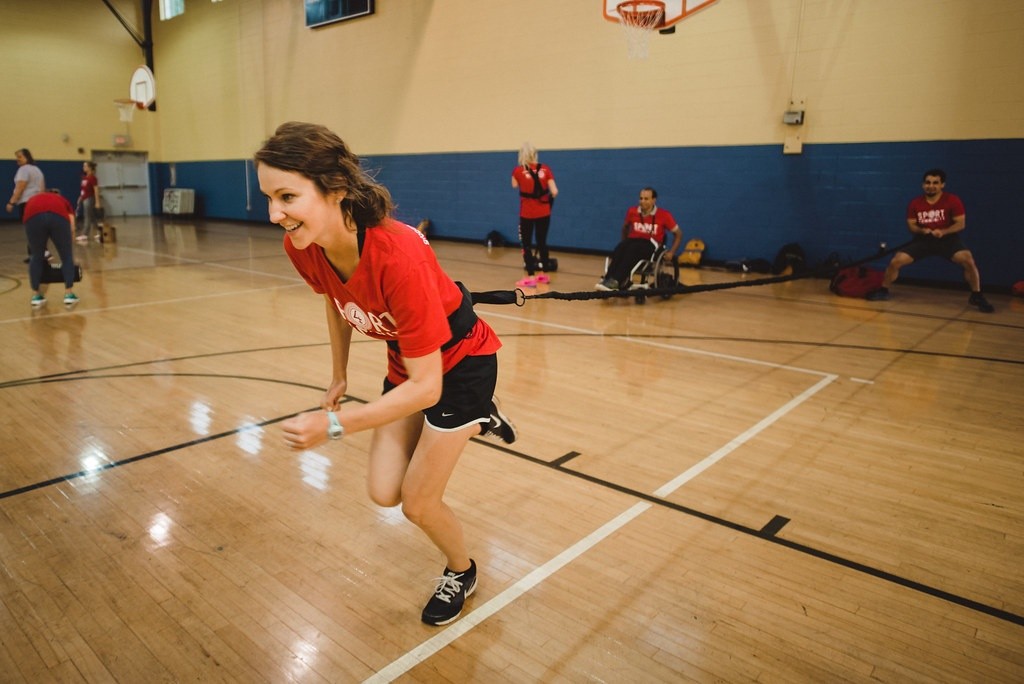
[24,189,79,303]
[7,148,55,263]
[76,161,101,240]
[596,188,680,291]
[864,170,995,312]
[254,120,519,627]
[512,143,558,275]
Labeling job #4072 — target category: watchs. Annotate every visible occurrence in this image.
[327,412,343,439]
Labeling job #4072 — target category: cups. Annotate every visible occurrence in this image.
[635,287,646,304]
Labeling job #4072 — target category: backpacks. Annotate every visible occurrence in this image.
[677,238,705,269]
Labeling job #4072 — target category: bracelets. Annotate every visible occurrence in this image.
[9,202,14,205]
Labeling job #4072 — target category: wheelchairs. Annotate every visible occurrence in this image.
[598,247,680,301]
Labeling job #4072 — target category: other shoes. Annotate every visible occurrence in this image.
[45,254,54,261]
[23,258,29,263]
[94,233,100,238]
[75,234,88,240]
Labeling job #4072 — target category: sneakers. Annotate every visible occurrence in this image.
[63,293,79,302]
[514,277,537,287]
[968,293,994,313]
[31,293,47,304]
[66,303,77,312]
[482,395,518,444]
[422,559,478,626]
[536,272,550,285]
[595,276,620,292]
[868,287,889,300]
[32,304,45,312]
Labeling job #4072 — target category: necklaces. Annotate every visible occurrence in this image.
[638,212,655,234]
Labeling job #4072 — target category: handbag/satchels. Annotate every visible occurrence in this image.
[725,243,852,278]
[829,267,881,296]
